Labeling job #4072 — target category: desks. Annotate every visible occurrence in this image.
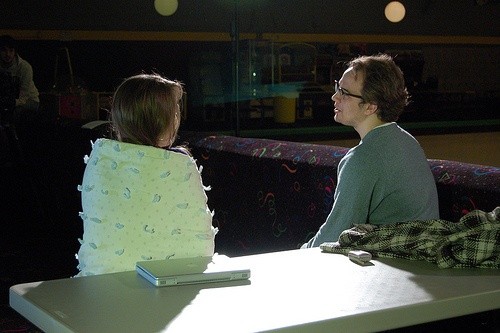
[9,247,500,333]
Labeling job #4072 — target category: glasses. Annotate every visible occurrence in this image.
[334,80,364,100]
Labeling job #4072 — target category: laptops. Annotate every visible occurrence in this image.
[136,254,250,287]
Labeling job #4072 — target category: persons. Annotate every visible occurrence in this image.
[72,74,220,277]
[0,34,40,112]
[300,55,439,246]
